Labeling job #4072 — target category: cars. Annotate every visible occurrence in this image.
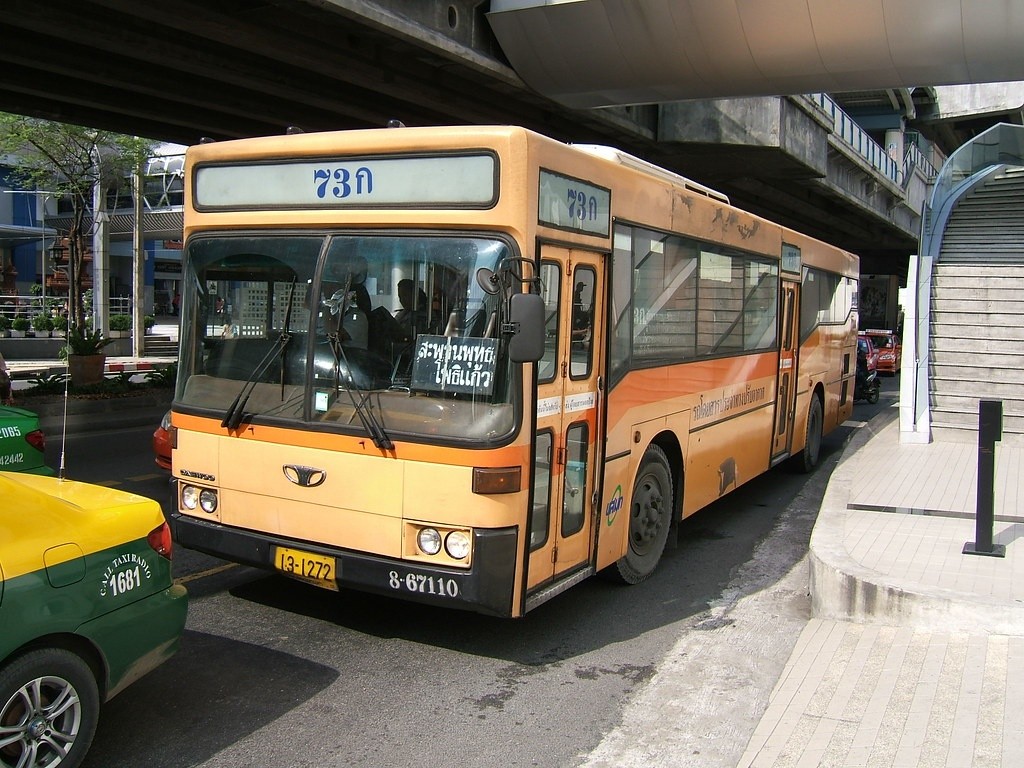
[0,402,57,478]
[857,330,879,373]
[0,467,190,768]
[865,328,902,377]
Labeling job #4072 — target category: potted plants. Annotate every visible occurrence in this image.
[108,315,154,338]
[51,316,68,337]
[0,316,12,338]
[12,316,32,338]
[60,324,115,387]
[33,315,54,337]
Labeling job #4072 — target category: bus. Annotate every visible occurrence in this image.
[165,120,859,621]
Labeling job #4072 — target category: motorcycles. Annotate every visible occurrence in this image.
[854,369,882,405]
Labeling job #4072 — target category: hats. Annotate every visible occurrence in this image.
[576,282,586,288]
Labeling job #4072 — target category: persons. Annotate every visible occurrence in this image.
[172,294,180,315]
[573,282,588,331]
[297,279,368,350]
[217,297,232,326]
[395,278,437,338]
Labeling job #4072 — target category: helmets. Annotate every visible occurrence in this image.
[858,341,863,350]
